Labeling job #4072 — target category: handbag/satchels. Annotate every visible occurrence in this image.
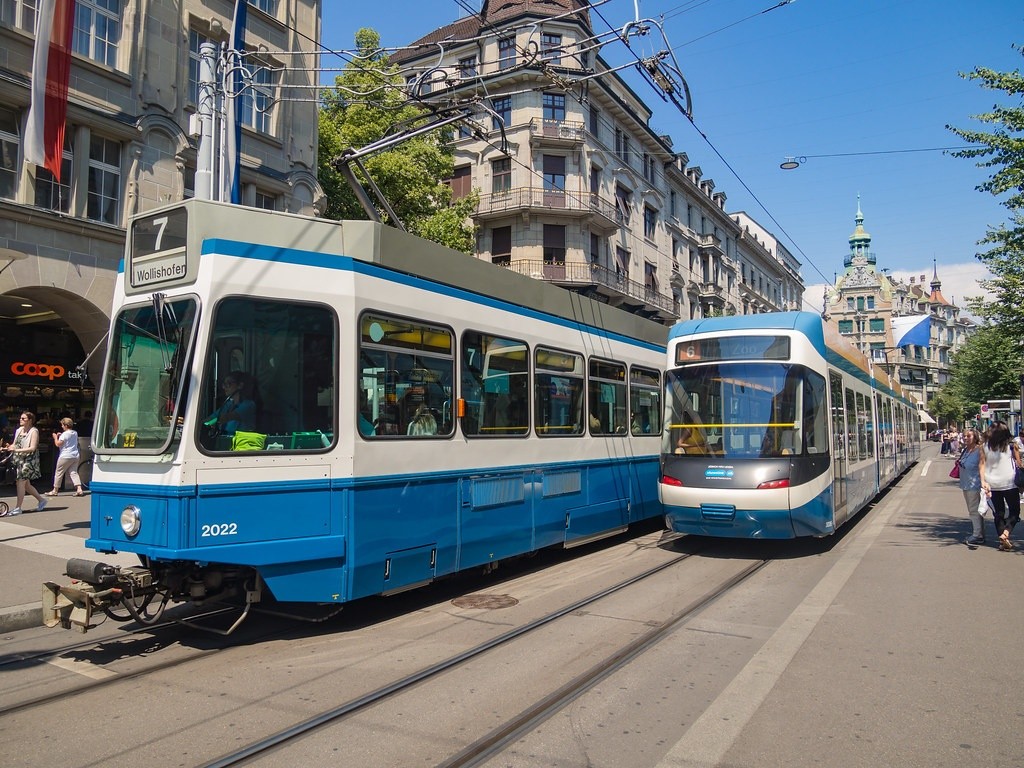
[977,488,995,523]
[950,447,967,478]
[1009,442,1024,487]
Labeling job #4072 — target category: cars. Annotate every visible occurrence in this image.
[928,428,950,443]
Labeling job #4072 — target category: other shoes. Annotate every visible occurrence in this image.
[73,491,85,496]
[966,535,984,546]
[45,490,58,496]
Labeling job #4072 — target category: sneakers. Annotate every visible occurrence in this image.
[7,507,22,516]
[37,499,48,511]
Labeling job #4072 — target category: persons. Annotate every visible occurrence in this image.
[0,412,48,516]
[678,414,708,455]
[303,362,642,436]
[941,421,1024,549]
[202,370,258,433]
[45,418,84,497]
[0,396,175,485]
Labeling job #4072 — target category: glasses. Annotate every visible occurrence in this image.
[222,382,239,389]
[20,417,29,420]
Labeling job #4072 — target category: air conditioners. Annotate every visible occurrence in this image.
[559,124,579,139]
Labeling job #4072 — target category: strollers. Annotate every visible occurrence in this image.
[0,448,14,518]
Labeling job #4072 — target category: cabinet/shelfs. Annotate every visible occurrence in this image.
[0,382,95,441]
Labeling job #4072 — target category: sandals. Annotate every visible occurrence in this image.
[999,534,1013,551]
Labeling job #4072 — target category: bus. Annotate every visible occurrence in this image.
[656,312,921,538]
[41,198,775,634]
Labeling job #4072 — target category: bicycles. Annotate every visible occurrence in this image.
[77,444,96,489]
